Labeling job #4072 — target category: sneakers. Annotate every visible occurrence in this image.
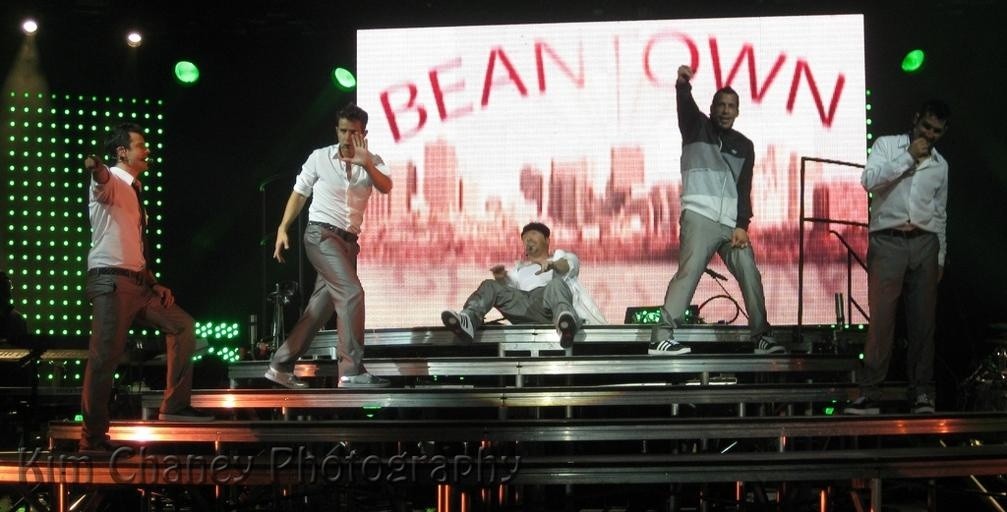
[264,364,310,390]
[337,372,391,389]
[159,402,216,422]
[78,439,136,460]
[441,308,474,343]
[648,337,691,356]
[910,392,935,414]
[754,336,786,355]
[556,310,578,349]
[844,396,880,415]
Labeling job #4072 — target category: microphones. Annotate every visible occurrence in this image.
[340,144,350,149]
[120,157,145,162]
[525,246,533,252]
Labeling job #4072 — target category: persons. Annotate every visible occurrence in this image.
[643,62,789,358]
[263,99,392,391]
[74,118,217,459]
[440,219,608,348]
[844,98,952,416]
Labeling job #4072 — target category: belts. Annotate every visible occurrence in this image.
[307,221,358,243]
[88,266,150,282]
[867,224,932,239]
